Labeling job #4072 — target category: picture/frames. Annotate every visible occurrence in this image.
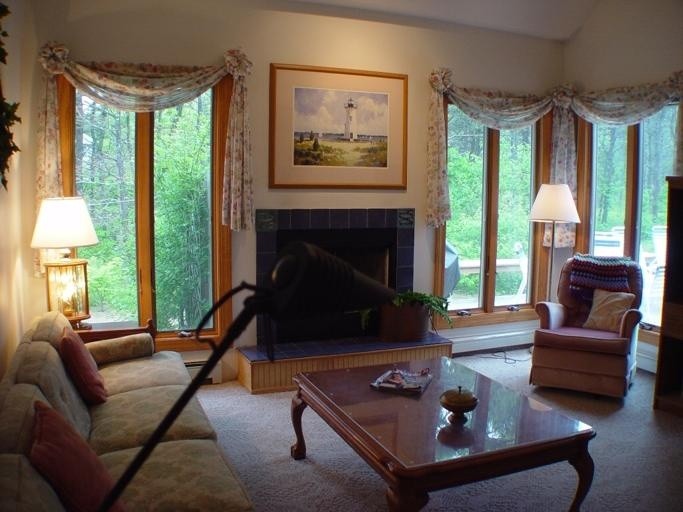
[269,62,409,190]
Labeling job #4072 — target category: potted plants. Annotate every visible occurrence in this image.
[361,290,453,343]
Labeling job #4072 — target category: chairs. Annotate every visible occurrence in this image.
[530,256,642,399]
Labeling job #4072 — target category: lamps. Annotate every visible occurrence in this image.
[29,197,100,330]
[527,184,581,300]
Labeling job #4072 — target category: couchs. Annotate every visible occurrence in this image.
[1,310,255,511]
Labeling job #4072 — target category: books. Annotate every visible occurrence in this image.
[369,368,434,395]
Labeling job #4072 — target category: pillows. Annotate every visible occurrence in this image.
[57,331,110,405]
[23,400,127,511]
[581,289,635,335]
